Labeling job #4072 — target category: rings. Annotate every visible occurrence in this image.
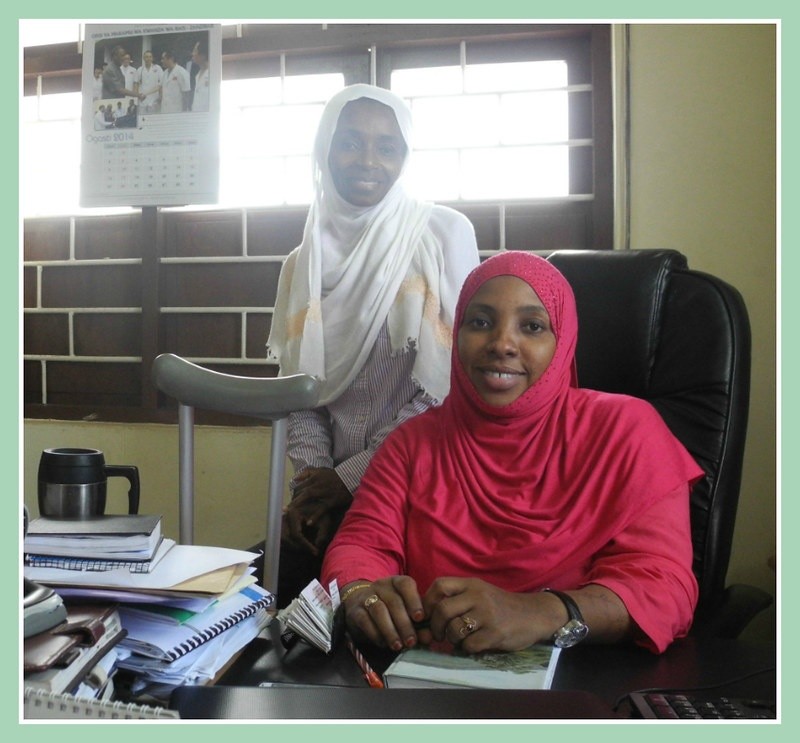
[365,595,378,607]
[459,615,477,636]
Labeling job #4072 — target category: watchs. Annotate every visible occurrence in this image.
[541,587,589,648]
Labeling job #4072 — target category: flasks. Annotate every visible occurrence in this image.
[37,447,140,520]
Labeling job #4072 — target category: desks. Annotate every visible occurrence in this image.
[119,610,777,719]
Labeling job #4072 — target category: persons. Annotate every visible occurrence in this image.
[183,60,200,112]
[102,45,139,99]
[191,40,209,112]
[246,84,480,610]
[133,50,163,115]
[94,65,105,99]
[143,50,192,114]
[322,252,705,656]
[120,52,137,92]
[95,100,137,131]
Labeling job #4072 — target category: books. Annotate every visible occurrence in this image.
[24,515,279,719]
[383,645,562,690]
[276,578,340,653]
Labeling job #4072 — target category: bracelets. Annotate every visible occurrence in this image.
[342,584,370,602]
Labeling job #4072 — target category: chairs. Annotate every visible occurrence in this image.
[542,249,751,591]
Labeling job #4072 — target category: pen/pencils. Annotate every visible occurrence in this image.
[343,631,383,688]
